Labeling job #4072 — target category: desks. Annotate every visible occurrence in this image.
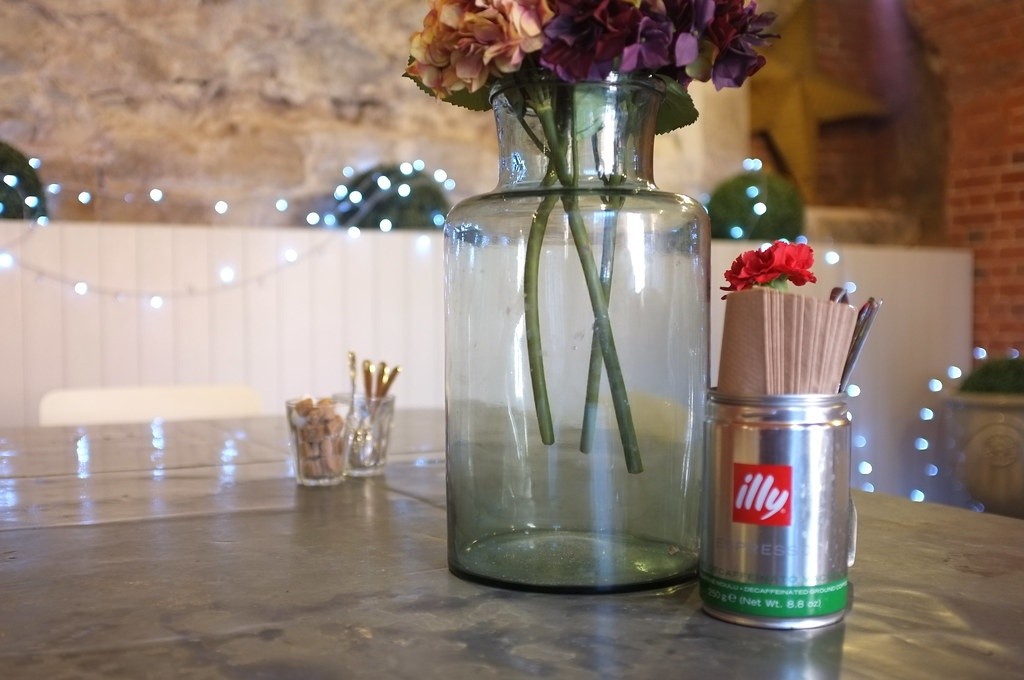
[0,399,1024,680]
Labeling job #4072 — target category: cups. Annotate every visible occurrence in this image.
[286,398,351,486]
[333,392,396,477]
[699,384,857,630]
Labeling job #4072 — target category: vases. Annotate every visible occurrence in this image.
[443,77,715,594]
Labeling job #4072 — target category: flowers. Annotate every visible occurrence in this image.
[719,239,817,304]
[404,0,780,472]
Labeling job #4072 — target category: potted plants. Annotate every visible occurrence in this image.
[946,359,1024,519]
